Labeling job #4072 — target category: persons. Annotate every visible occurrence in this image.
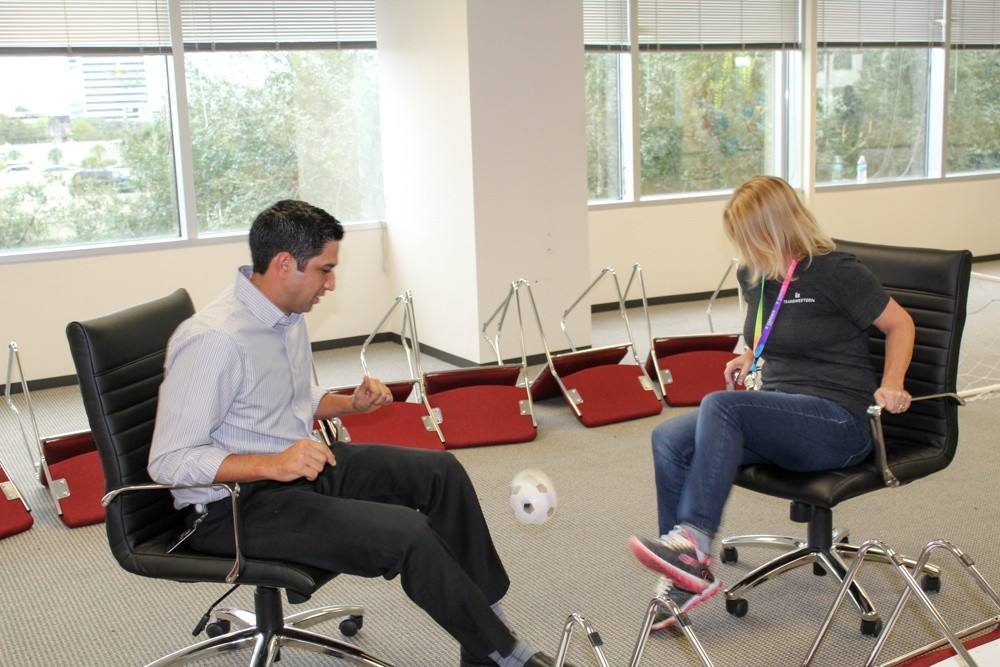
[148,199,577,667]
[628,174,916,628]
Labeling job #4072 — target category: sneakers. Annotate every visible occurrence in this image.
[649,569,721,631]
[626,526,715,595]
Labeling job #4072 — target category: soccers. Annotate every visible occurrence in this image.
[508,470,559,523]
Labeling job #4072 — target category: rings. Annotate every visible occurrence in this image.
[898,404,904,411]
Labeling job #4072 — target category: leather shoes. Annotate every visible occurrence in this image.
[483,649,556,667]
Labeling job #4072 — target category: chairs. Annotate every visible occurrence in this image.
[401,279,542,449]
[301,297,445,459]
[66,287,396,667]
[482,237,1000,667]
[0,341,106,541]
